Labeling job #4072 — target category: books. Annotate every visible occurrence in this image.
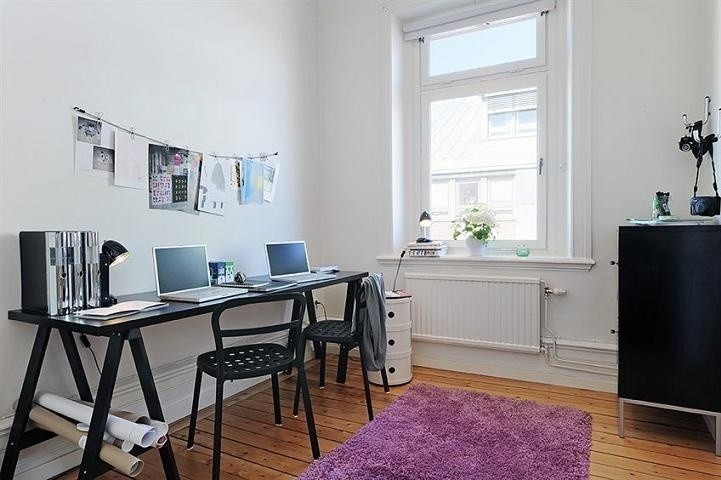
[73,301,170,321]
[19,230,101,315]
[220,280,269,287]
[247,282,298,292]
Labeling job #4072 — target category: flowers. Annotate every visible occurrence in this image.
[450,202,504,247]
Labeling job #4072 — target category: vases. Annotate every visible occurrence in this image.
[464,239,485,257]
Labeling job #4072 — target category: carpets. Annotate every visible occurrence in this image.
[292,380,594,480]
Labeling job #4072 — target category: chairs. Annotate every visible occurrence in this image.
[293,283,390,422]
[185,291,322,479]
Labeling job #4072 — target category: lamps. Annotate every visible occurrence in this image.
[416,209,434,243]
[97,238,132,308]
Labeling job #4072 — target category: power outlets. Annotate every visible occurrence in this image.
[313,298,319,311]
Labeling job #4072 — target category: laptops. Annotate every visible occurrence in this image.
[265,241,337,283]
[151,244,248,303]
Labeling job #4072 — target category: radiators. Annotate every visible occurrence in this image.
[403,271,547,356]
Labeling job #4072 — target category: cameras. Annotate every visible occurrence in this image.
[688,193,719,217]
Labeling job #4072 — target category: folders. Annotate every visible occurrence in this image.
[21,228,101,313]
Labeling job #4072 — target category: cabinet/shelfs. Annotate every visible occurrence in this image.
[609,222,721,458]
[362,297,413,387]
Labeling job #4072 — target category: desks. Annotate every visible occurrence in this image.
[1,267,370,478]
